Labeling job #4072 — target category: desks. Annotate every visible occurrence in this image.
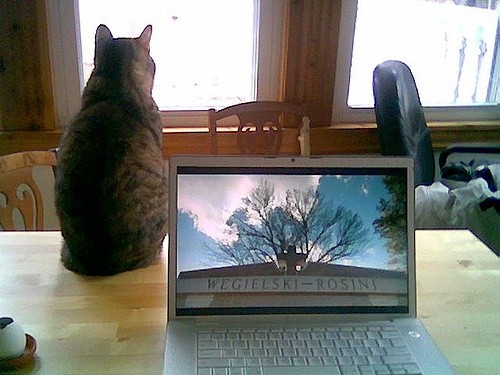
[0,228,500,375]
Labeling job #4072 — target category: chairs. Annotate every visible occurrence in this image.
[1,151,61,231]
[208,100,311,155]
[374,59,500,230]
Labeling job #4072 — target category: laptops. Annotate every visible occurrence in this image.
[162,154,458,375]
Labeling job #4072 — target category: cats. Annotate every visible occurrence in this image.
[53,23,170,278]
